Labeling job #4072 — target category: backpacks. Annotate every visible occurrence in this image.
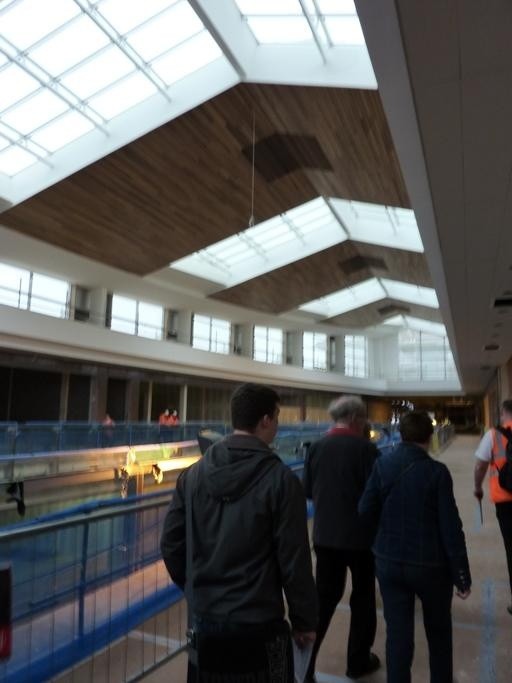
[493,426,512,495]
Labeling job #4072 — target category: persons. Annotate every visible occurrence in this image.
[170,407,180,442]
[159,407,170,443]
[301,394,385,679]
[101,410,116,447]
[157,381,323,683]
[473,398,512,615]
[357,408,473,683]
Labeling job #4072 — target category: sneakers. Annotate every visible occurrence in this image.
[346,652,379,678]
